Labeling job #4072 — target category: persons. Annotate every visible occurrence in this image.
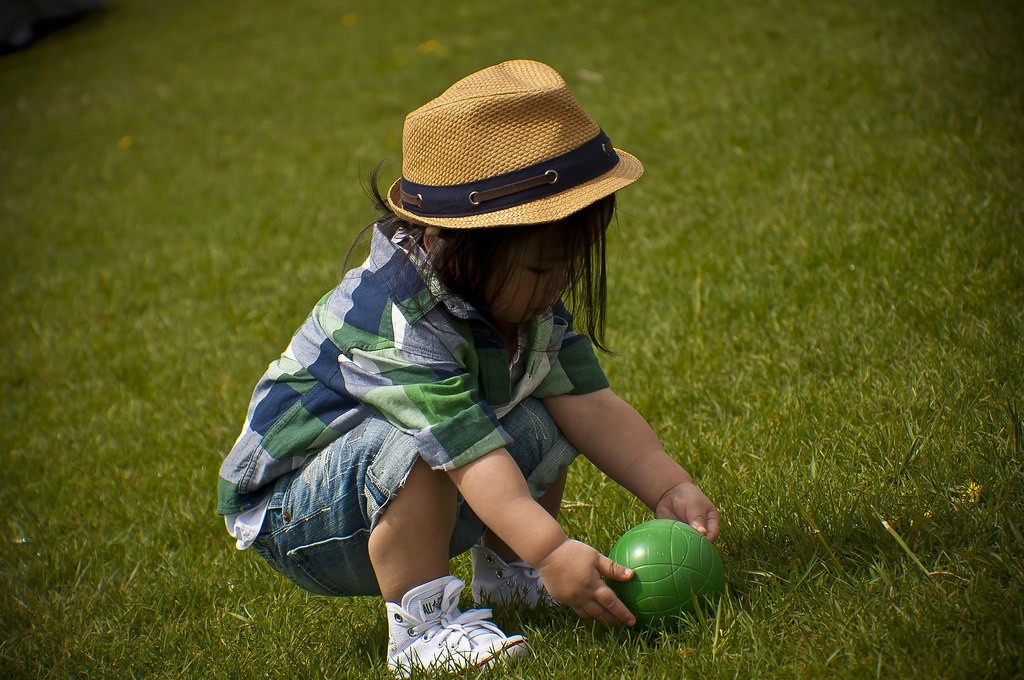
[218,56,722,680]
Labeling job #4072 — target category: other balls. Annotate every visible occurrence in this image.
[607,517,728,637]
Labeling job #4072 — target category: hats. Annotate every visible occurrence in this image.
[387,58,645,229]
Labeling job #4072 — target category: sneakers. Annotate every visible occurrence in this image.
[469,539,569,619]
[385,576,531,679]
